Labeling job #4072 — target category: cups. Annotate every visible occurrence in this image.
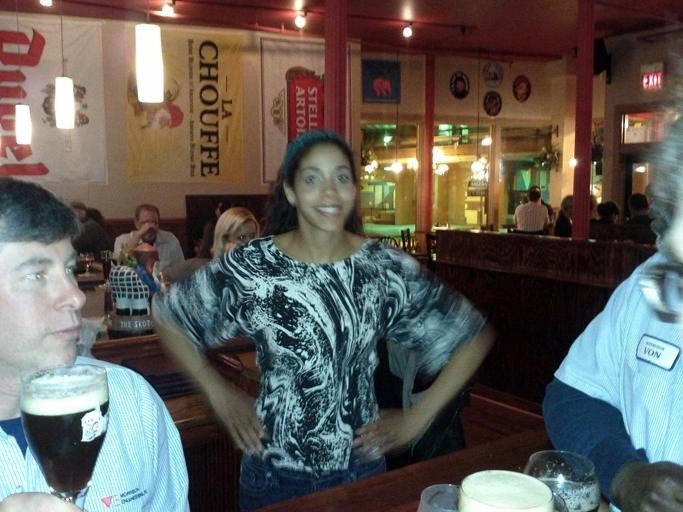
[416,449,603,511]
[102,250,114,281]
[102,285,113,316]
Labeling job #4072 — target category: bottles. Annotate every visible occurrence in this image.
[143,227,156,247]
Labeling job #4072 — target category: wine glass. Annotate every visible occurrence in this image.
[77,251,99,278]
[16,360,110,512]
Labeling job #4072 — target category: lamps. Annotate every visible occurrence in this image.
[15,0,165,146]
[39,0,461,38]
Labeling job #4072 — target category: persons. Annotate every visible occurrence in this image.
[151,130,498,512]
[542,40,683,512]
[513,186,658,246]
[0,175,191,512]
[68,201,261,286]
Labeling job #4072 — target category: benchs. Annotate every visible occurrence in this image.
[92,333,256,431]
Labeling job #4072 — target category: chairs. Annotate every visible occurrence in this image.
[380,228,437,272]
